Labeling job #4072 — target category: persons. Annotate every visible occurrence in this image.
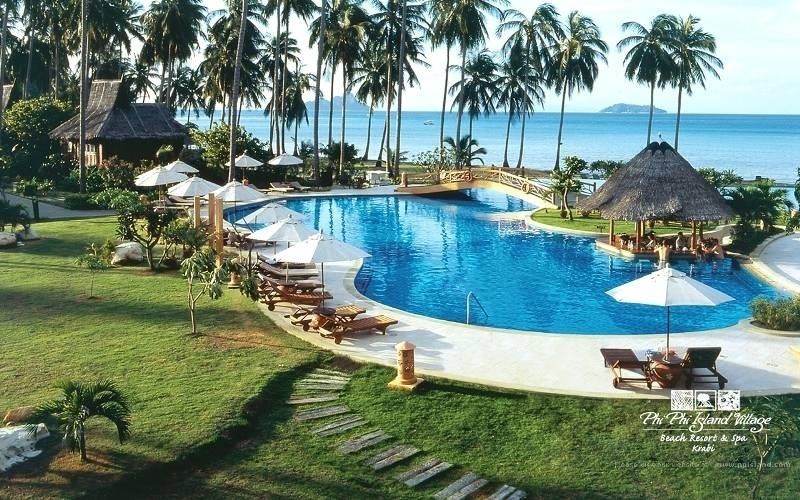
[620,231,724,271]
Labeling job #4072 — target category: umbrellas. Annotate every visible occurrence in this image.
[134,152,315,282]
[604,262,736,360]
[273,229,373,312]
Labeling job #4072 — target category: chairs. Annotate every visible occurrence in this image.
[599,346,729,392]
[223,232,400,345]
[248,179,312,194]
